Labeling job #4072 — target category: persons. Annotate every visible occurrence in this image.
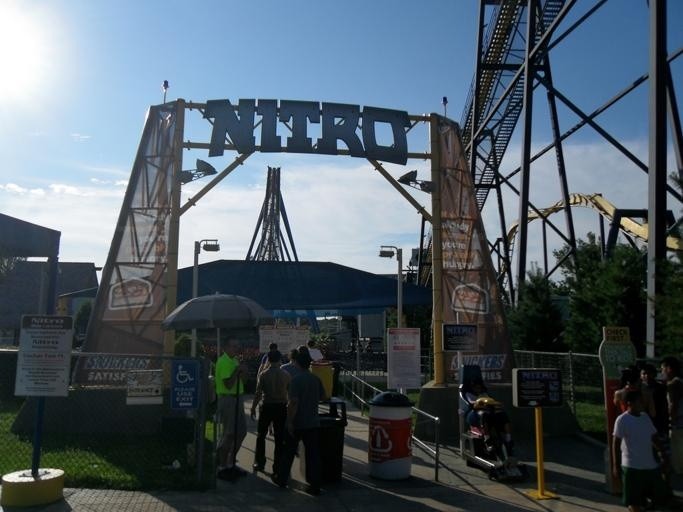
[659,356,683,477]
[640,364,673,484]
[610,391,673,511]
[611,369,643,413]
[464,376,491,428]
[213,336,325,486]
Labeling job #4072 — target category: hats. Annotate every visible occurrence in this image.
[297,345,312,370]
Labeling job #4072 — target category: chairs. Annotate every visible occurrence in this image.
[458,363,493,434]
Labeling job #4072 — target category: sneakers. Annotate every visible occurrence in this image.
[271,472,289,490]
[252,462,266,471]
[301,483,321,497]
[218,465,249,483]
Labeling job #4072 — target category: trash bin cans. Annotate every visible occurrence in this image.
[300,397,347,488]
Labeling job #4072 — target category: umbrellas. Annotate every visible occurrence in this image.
[159,289,275,359]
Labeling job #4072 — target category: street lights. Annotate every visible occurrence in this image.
[379,246,402,329]
[190,239,219,360]
